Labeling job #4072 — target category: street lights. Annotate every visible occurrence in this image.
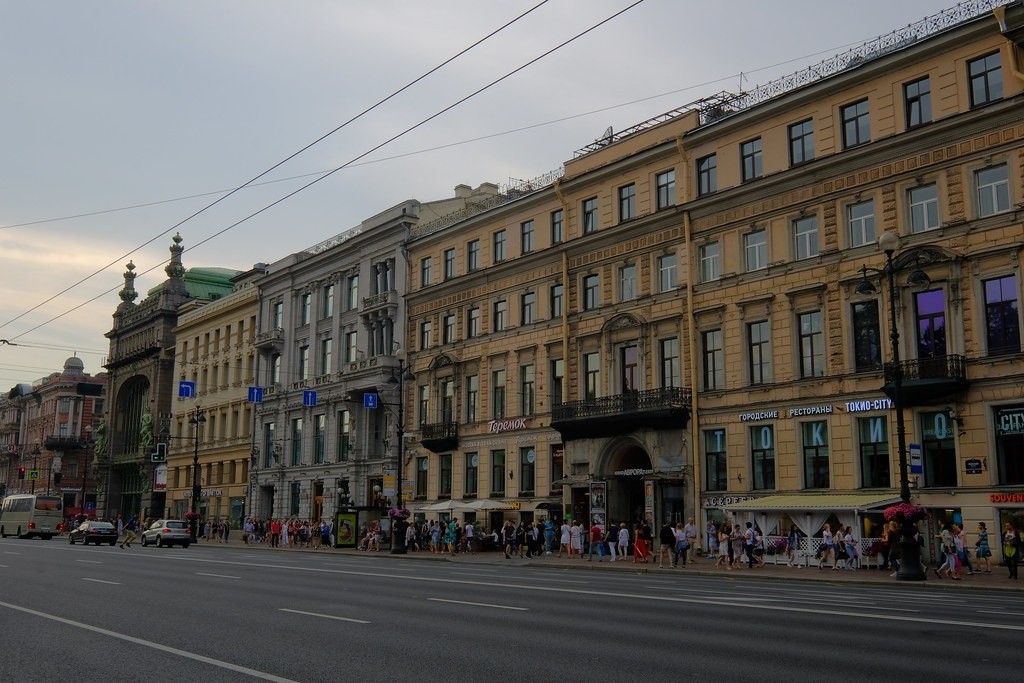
[78,424,97,524]
[30,437,43,495]
[854,232,932,582]
[385,348,416,555]
[188,406,207,543]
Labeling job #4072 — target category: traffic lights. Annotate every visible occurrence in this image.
[152,443,166,463]
[18,467,25,479]
[194,485,201,501]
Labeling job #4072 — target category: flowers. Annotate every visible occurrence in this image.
[185,512,201,521]
[863,539,886,558]
[883,501,931,523]
[765,537,788,556]
[389,509,411,520]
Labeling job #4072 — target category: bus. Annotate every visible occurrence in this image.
[0,493,64,540]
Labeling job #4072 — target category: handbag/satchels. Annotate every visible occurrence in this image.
[944,545,956,553]
[785,549,790,559]
[974,546,978,558]
[819,543,827,551]
[918,532,924,547]
[745,544,753,551]
[958,551,964,560]
[686,544,690,549]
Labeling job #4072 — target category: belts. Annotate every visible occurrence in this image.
[689,537,696,538]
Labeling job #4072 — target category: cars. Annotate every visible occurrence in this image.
[69,520,118,546]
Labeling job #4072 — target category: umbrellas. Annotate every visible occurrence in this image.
[456,499,520,529]
[419,500,466,522]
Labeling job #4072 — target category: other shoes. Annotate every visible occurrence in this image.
[501,551,862,569]
[879,562,1018,581]
[126,543,130,547]
[120,545,124,549]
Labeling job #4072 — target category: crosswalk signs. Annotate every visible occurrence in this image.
[29,471,38,480]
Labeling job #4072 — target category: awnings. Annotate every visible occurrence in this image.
[720,495,906,512]
[536,503,562,510]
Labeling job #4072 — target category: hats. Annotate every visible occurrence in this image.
[573,520,577,522]
[620,523,625,527]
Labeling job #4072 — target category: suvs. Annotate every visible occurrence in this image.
[140,519,191,548]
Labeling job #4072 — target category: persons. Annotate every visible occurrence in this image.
[592,487,604,508]
[93,418,108,453]
[1000,521,1021,580]
[786,525,802,568]
[592,514,601,527]
[140,406,153,446]
[879,521,929,577]
[73,513,383,552]
[647,512,653,533]
[817,523,860,572]
[933,518,992,580]
[403,516,765,571]
